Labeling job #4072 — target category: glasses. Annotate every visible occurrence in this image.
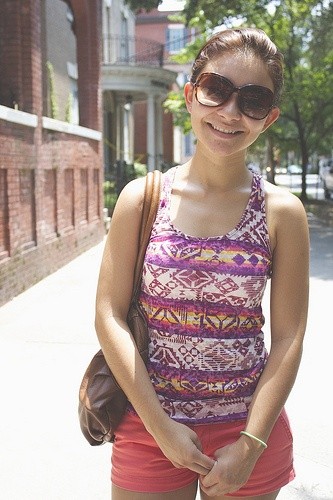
[192,72,274,120]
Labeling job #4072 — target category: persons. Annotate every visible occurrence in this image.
[94,27,310,500]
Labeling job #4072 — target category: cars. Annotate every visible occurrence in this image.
[319,157,333,199]
[262,164,304,175]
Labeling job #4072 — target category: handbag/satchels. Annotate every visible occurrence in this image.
[78,169,162,447]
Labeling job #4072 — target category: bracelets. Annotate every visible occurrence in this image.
[240,430,267,448]
